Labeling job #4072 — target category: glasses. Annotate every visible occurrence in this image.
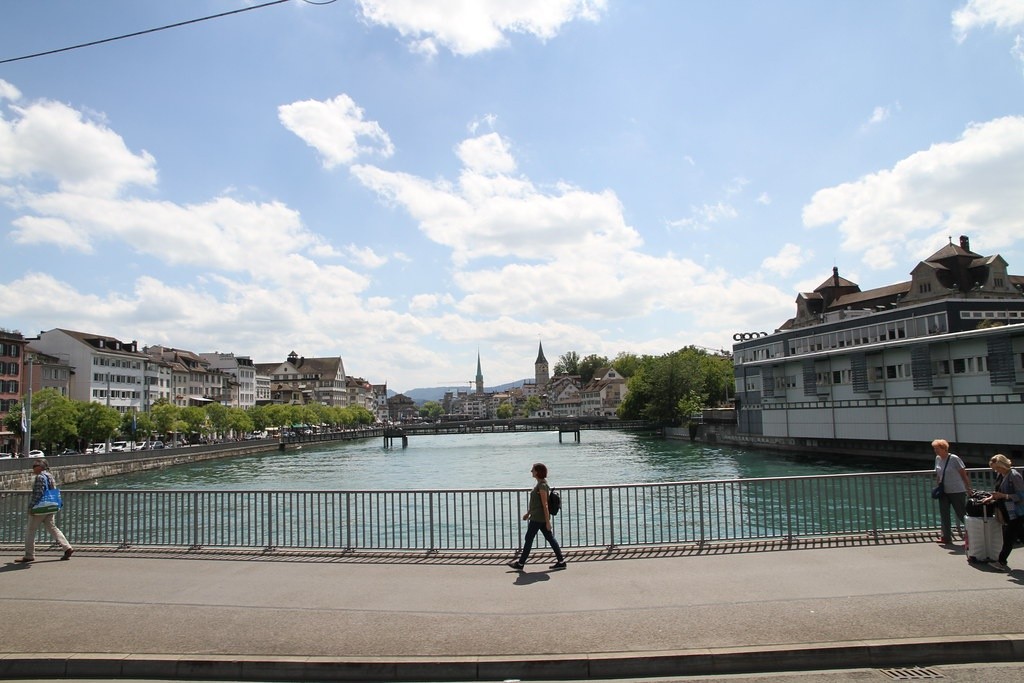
[32,463,41,468]
[530,469,536,472]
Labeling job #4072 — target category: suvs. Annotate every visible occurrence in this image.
[85,441,182,454]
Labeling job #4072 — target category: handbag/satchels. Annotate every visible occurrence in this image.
[29,488,62,516]
[931,481,945,499]
[1006,514,1024,549]
[965,489,997,516]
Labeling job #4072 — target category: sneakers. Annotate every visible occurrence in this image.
[548,562,567,571]
[61,548,75,560]
[936,537,953,543]
[988,561,1006,571]
[15,556,35,562]
[507,561,524,570]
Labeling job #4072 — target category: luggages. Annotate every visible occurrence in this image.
[964,503,1003,561]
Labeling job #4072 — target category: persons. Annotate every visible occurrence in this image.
[506,463,566,571]
[14,458,74,563]
[932,439,975,549]
[988,454,1024,571]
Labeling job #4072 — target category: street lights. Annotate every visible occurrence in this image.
[23,356,33,457]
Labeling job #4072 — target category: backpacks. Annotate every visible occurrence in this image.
[540,480,562,516]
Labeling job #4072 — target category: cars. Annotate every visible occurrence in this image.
[59,448,78,455]
[0,452,24,460]
[29,449,45,457]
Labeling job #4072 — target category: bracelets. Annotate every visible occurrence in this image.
[1006,494,1008,499]
